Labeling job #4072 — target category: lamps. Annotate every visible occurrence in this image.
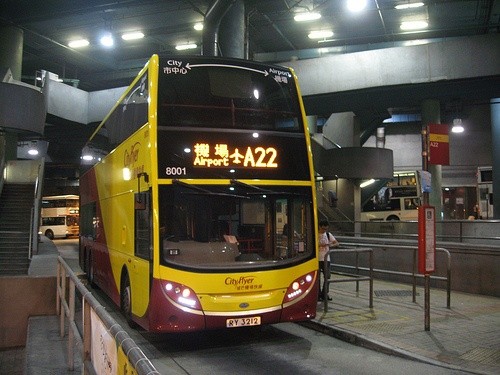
[28,139,39,156]
[451,101,465,134]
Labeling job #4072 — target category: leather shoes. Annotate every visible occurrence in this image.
[318,296,332,301]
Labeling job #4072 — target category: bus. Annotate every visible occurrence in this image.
[359,185,420,233]
[78,53,320,335]
[39,194,79,240]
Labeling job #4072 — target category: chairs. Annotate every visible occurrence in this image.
[207,219,230,243]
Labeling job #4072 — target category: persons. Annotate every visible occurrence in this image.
[318,220,340,302]
